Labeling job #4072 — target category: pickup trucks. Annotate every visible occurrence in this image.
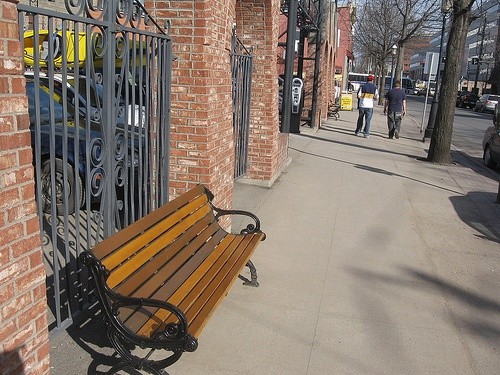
[456,90,480,108]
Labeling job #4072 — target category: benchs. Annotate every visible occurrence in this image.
[328,99,341,121]
[79,183,266,375]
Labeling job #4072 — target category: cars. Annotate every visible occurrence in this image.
[25,69,149,217]
[481,125,500,171]
[417,88,436,97]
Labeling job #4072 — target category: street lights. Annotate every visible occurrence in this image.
[390,44,398,89]
[422,0,453,142]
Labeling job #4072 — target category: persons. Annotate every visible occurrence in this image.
[355,75,379,138]
[384,80,406,139]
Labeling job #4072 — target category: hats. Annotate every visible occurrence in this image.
[367,75,373,81]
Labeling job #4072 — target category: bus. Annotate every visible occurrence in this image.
[348,72,415,95]
[414,80,426,95]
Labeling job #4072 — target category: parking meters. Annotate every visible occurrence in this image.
[289,75,304,133]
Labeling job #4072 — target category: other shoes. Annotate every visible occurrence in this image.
[389,136,393,139]
[395,129,399,139]
[364,134,369,138]
[355,131,359,135]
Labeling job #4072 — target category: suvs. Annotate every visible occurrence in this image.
[474,94,500,112]
